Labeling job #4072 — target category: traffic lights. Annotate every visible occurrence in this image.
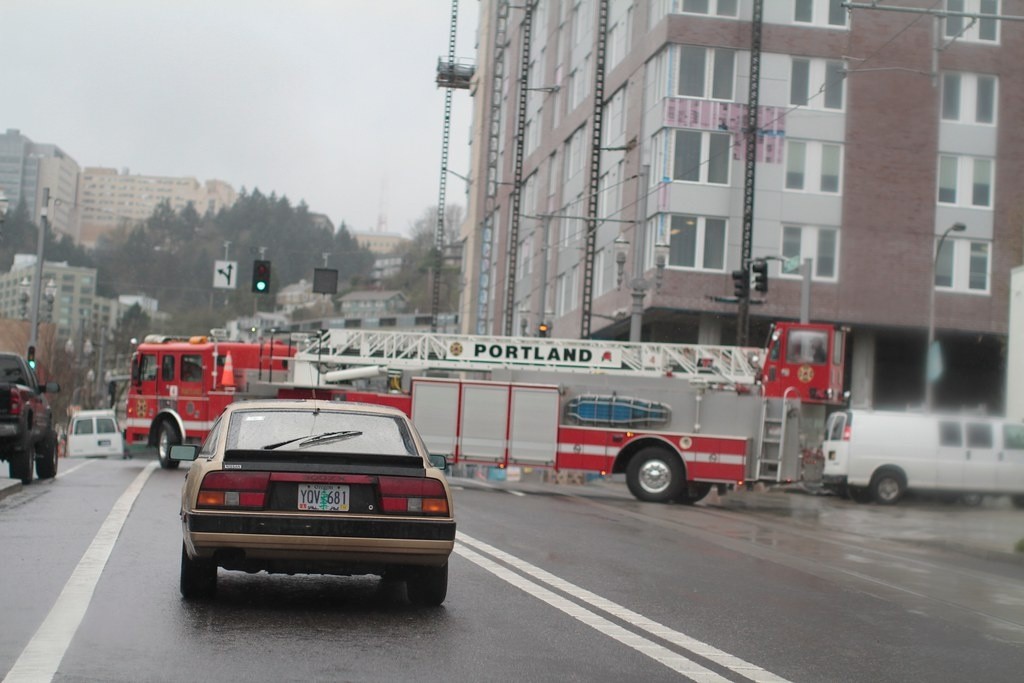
[731,268,747,298]
[752,262,768,294]
[250,259,272,295]
[27,346,36,369]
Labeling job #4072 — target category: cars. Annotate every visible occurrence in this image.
[176,396,459,611]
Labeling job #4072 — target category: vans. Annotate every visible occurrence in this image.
[63,409,124,460]
[817,410,1023,507]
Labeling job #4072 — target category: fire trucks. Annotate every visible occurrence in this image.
[114,320,850,505]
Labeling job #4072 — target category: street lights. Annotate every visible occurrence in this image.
[917,221,972,407]
[14,275,103,407]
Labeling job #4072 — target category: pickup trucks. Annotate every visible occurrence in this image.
[0,351,59,487]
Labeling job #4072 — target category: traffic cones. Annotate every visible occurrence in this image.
[217,350,238,386]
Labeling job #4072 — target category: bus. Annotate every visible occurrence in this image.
[108,372,150,445]
